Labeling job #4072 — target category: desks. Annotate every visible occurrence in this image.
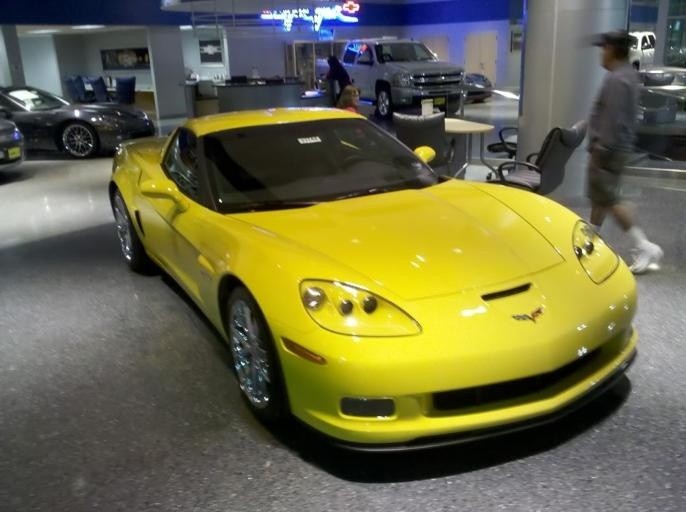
[443,118,498,179]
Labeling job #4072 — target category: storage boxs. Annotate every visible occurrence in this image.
[194,97,219,115]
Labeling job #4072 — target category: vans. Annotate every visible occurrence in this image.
[627,31,658,72]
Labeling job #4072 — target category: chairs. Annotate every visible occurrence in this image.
[393,111,456,177]
[484,126,585,195]
[486,127,517,160]
[63,72,136,110]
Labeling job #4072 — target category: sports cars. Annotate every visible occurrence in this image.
[107,104,639,455]
[463,72,494,105]
[0,84,156,160]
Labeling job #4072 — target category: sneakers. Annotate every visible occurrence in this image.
[629,241,663,274]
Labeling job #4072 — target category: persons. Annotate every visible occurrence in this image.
[320,54,351,107]
[582,27,665,277]
[335,84,365,137]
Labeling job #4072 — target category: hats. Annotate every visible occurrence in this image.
[591,31,637,46]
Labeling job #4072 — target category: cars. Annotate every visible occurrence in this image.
[0,118,25,165]
[633,68,686,140]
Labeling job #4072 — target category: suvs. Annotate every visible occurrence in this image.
[334,37,465,120]
